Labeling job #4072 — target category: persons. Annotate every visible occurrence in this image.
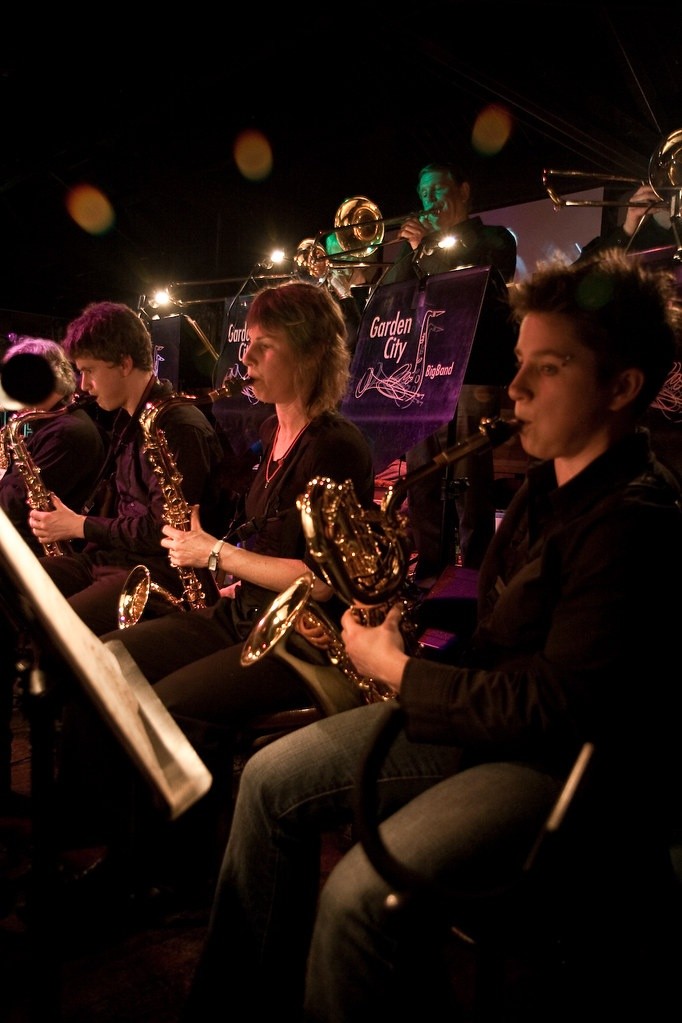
[0,338,105,556]
[182,249,681,1023]
[570,185,682,267]
[18,301,230,689]
[326,261,380,352]
[57,278,374,916]
[385,160,518,601]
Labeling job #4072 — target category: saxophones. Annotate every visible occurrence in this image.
[239,410,528,704]
[117,361,247,646]
[2,387,93,566]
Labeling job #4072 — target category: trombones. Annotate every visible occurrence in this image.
[167,237,352,306]
[538,128,682,240]
[312,194,447,270]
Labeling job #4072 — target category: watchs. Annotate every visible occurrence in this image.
[208,540,225,571]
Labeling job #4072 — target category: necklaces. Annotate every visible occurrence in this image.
[264,422,310,488]
